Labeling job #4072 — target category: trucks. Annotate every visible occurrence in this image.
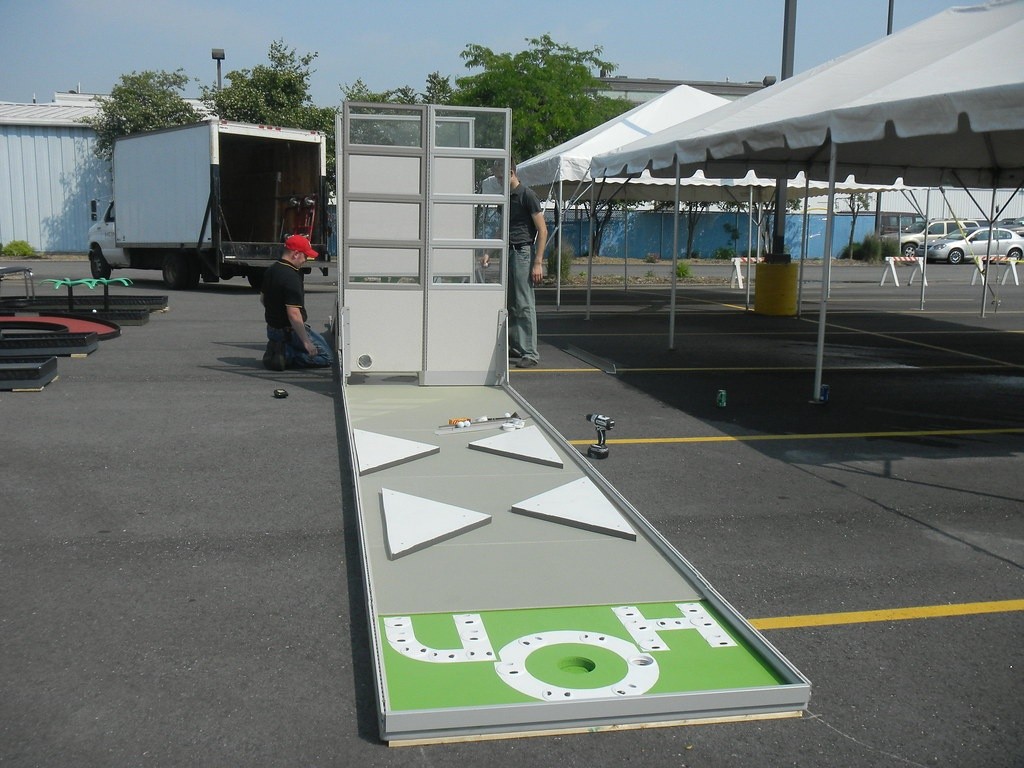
[86,119,328,291]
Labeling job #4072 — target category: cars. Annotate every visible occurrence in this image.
[997,216,1024,235]
[918,226,1024,265]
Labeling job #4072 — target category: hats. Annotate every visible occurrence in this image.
[284,235,319,259]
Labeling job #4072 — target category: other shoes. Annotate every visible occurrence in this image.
[516,357,538,368]
[263,340,275,369]
[509,349,522,358]
[271,342,285,371]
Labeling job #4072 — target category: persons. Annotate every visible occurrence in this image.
[481,159,548,367]
[261,235,334,370]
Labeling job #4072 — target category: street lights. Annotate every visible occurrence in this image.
[210,48,226,100]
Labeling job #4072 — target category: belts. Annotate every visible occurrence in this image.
[510,242,534,250]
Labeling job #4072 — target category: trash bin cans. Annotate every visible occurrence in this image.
[755,253,799,316]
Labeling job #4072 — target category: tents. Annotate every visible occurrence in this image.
[481,84,931,310]
[586,0,1024,405]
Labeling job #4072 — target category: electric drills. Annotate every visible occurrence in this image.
[582,413,615,459]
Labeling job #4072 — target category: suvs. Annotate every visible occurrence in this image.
[878,218,981,260]
